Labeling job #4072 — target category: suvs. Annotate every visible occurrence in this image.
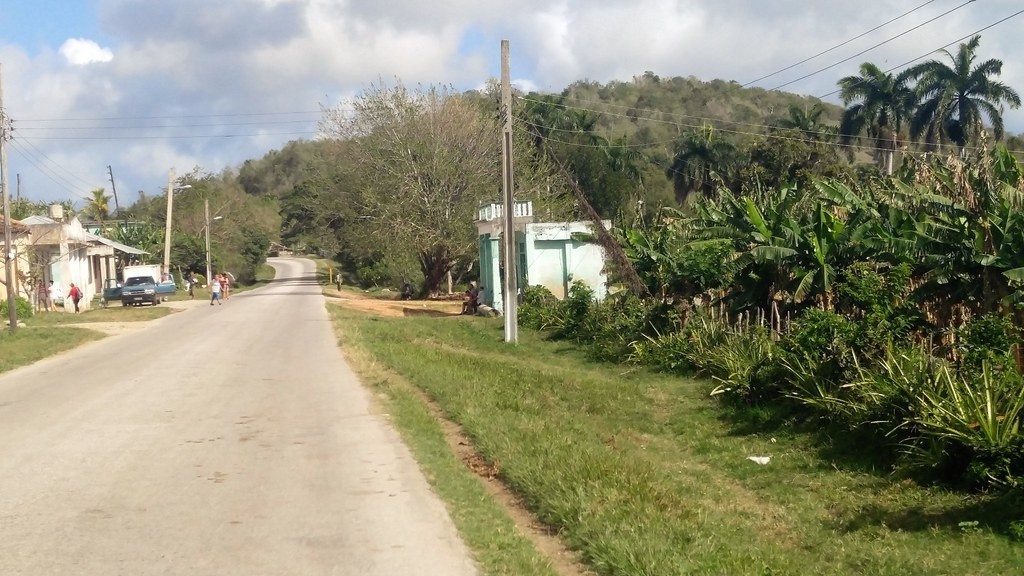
[103,273,176,306]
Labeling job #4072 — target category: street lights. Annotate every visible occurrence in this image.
[164,185,192,301]
[206,216,223,286]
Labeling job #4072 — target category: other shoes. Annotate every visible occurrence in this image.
[457,313,462,315]
[210,303,214,305]
[463,311,467,314]
[219,302,221,305]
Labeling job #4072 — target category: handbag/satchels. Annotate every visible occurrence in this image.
[76,287,83,299]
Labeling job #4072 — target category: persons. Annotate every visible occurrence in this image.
[209,275,225,305]
[469,284,478,296]
[47,280,58,311]
[335,272,343,291]
[219,273,229,300]
[461,290,477,315]
[470,286,486,314]
[188,272,198,300]
[400,284,412,300]
[67,283,81,313]
[36,280,49,311]
[163,273,168,282]
